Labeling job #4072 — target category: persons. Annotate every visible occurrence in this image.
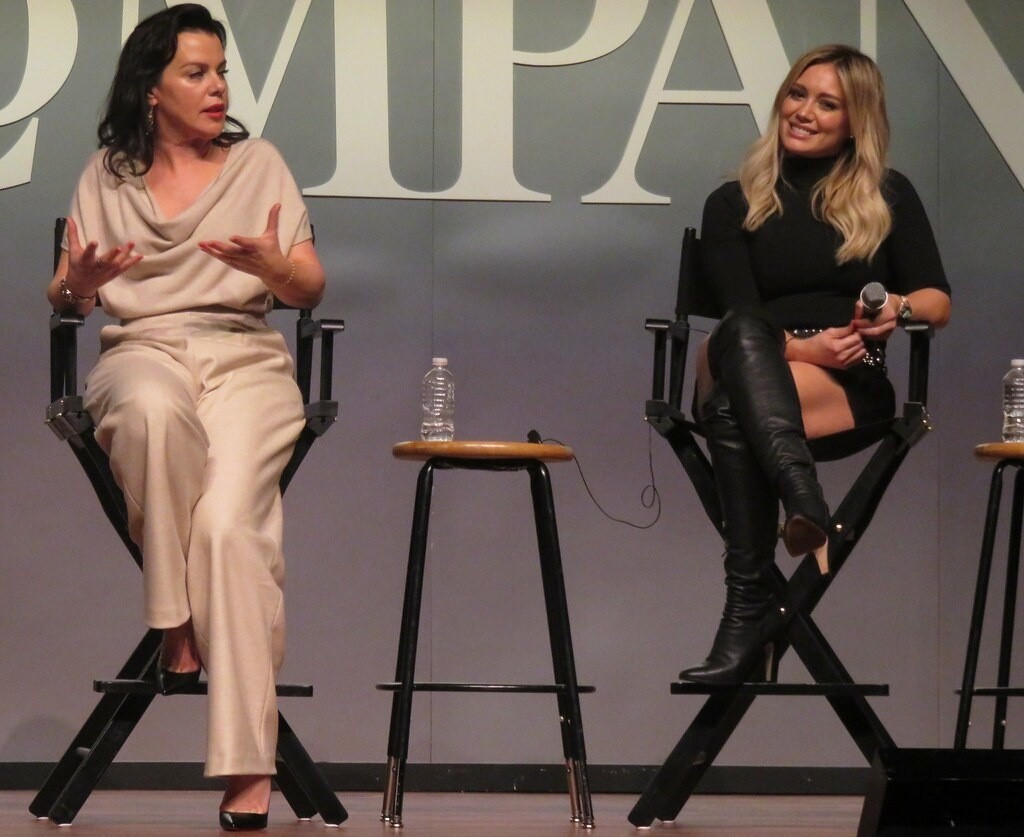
[674,46,951,685]
[46,3,323,832]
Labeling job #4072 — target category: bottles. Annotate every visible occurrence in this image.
[1002,359,1024,442]
[420,358,456,441]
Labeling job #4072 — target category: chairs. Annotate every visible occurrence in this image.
[625,226,897,831]
[27,217,349,829]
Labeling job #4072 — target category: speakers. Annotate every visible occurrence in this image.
[856,746,1024,837]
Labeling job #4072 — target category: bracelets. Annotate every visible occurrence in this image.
[97,260,108,270]
[58,277,97,307]
[262,259,295,292]
[226,258,230,263]
[897,295,912,324]
[785,336,796,344]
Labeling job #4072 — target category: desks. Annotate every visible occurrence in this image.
[382,439,596,831]
[953,443,1024,749]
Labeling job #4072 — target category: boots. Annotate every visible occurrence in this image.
[707,308,833,575]
[679,378,780,684]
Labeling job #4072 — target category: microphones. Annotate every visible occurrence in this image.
[860,281,889,323]
[527,429,544,446]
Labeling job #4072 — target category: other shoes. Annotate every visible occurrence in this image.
[154,621,202,696]
[218,776,273,831]
